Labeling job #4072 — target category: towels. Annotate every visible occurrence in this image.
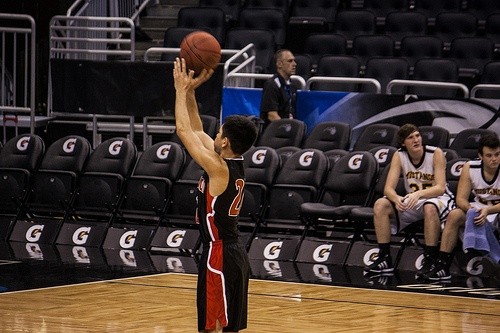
[463,207,500,264]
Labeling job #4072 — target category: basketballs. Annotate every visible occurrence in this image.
[180,31,221,74]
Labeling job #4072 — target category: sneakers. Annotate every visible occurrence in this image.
[423,259,452,280]
[415,253,439,280]
[365,254,395,272]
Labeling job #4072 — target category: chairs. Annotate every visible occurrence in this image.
[0,0,500,282]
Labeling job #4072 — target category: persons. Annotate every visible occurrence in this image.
[428,138,500,280]
[169,56,259,333]
[363,123,457,275]
[259,48,297,135]
